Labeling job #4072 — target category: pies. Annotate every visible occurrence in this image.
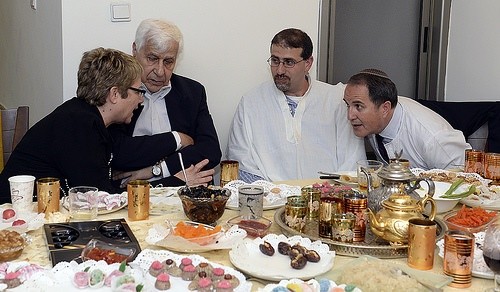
[340,175,357,183]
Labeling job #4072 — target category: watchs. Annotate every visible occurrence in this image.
[151,165,162,177]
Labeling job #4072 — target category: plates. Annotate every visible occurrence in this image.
[158,222,226,253]
[229,240,335,281]
[0,259,359,292]
[225,185,287,211]
[412,167,500,280]
[0,194,127,232]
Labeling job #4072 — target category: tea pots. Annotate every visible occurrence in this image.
[366,191,436,246]
[359,157,435,212]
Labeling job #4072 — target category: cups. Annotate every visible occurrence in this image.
[127,180,150,220]
[36,178,60,214]
[443,230,475,289]
[285,160,383,243]
[464,150,484,175]
[68,186,98,221]
[219,160,239,189]
[8,174,36,214]
[485,153,500,180]
[237,186,264,215]
[408,218,436,270]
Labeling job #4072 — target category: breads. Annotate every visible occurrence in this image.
[0,230,24,262]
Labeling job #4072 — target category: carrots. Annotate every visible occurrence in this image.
[447,204,497,232]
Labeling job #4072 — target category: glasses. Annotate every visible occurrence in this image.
[267,57,305,68]
[128,87,145,97]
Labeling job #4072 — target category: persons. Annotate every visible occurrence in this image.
[227,29,369,185]
[0,48,214,204]
[342,70,471,170]
[106,18,221,189]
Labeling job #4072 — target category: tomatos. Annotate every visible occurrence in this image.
[12,220,25,226]
[3,209,15,220]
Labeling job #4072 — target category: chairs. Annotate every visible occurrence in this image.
[415,101,500,153]
[0,106,29,172]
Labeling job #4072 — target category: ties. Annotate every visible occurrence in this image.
[374,133,390,165]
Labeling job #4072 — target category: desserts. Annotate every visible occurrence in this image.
[145,257,240,292]
[73,268,138,292]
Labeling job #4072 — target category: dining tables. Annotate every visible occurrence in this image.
[1,178,500,292]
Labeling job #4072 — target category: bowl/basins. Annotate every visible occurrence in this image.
[177,184,272,245]
[412,180,460,214]
[442,208,499,237]
[226,214,272,238]
[0,228,35,261]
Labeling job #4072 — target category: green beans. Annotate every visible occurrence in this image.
[441,178,477,199]
